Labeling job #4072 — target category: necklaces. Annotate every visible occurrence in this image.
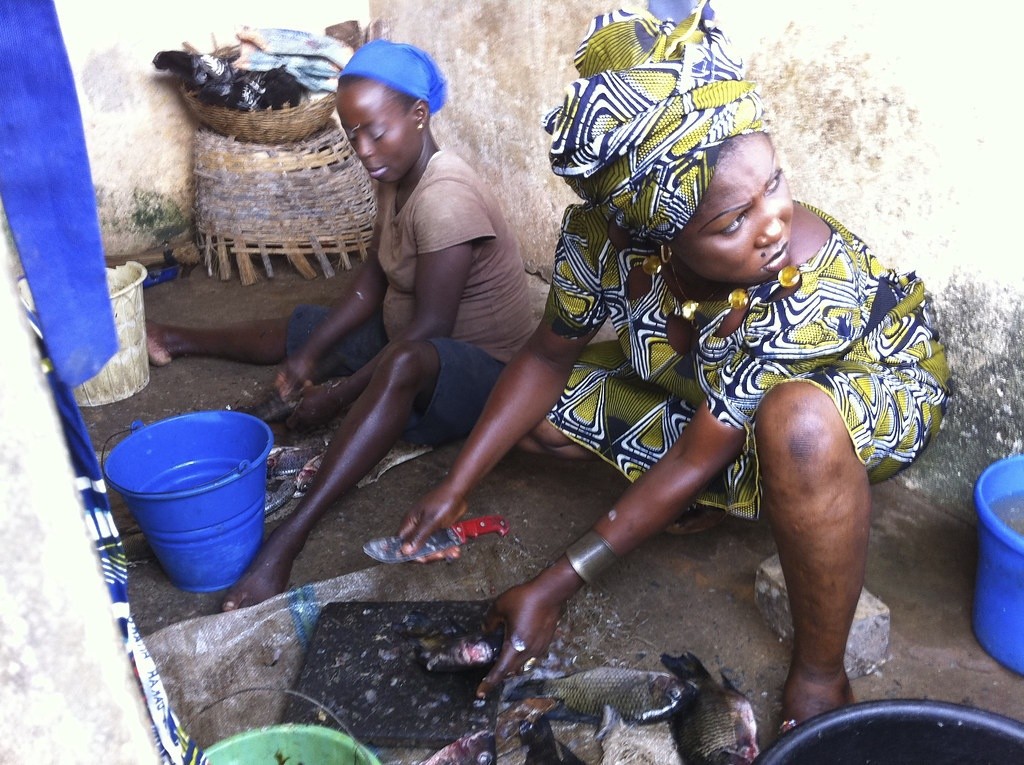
[668,257,723,302]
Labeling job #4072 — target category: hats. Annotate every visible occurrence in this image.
[340,41,445,113]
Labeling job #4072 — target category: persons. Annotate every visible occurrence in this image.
[395,0,948,740]
[144,39,533,613]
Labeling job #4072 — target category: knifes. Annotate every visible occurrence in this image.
[363,515,510,564]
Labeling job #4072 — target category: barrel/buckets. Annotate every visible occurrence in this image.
[18,260,152,406]
[102,408,273,592]
[184,684,381,765]
[972,454,1023,677]
[750,699,1024,764]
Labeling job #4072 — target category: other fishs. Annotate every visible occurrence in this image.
[392,609,505,672]
[423,652,760,765]
[251,379,329,515]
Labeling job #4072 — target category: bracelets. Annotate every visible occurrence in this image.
[324,381,346,410]
[564,528,619,587]
